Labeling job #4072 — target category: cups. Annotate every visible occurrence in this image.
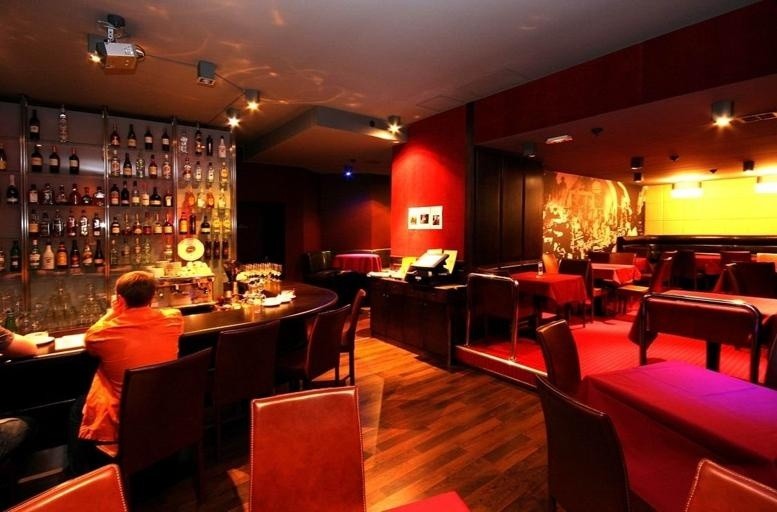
[240,296,266,314]
[538,262,543,276]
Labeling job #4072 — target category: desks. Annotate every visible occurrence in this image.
[332,252,382,274]
[368,269,467,367]
[576,361,777,511]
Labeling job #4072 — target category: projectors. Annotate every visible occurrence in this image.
[96,41,137,71]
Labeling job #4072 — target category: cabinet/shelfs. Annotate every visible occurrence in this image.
[0,92,238,337]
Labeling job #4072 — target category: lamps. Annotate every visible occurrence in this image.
[387,116,401,135]
[521,142,537,158]
[630,156,643,182]
[711,99,734,123]
[196,60,260,128]
[743,161,754,173]
[95,13,144,71]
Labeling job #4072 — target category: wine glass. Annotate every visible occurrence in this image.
[0,276,107,333]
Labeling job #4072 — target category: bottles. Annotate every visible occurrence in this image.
[0,145,21,273]
[28,105,105,271]
[179,125,232,260]
[223,265,232,303]
[109,121,173,266]
[236,263,282,293]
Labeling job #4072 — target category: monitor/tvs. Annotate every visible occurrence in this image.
[411,254,449,268]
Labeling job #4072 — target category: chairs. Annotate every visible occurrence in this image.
[117,345,214,499]
[531,372,630,512]
[213,320,282,461]
[248,385,367,511]
[307,288,368,386]
[682,459,777,511]
[278,303,352,390]
[2,462,129,512]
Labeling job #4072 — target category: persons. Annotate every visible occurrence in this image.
[433,215,439,225]
[78,270,184,448]
[0,326,39,490]
[420,214,427,224]
[410,216,416,225]
